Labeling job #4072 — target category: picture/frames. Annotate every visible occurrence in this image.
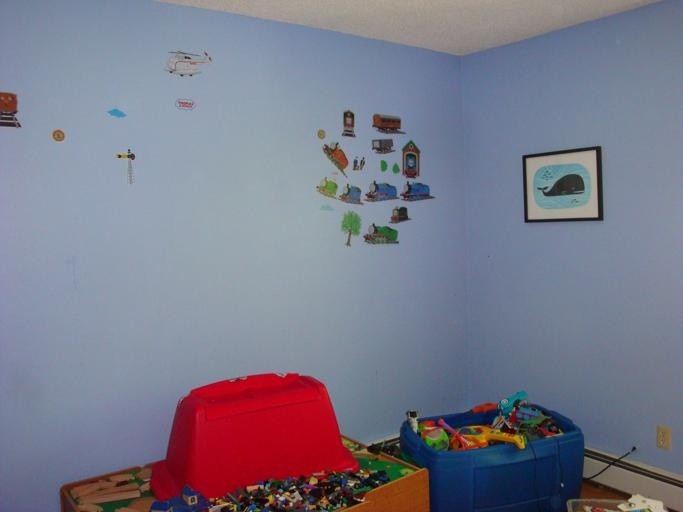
[521,145,604,223]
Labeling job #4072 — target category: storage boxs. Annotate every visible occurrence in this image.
[399,402,586,511]
